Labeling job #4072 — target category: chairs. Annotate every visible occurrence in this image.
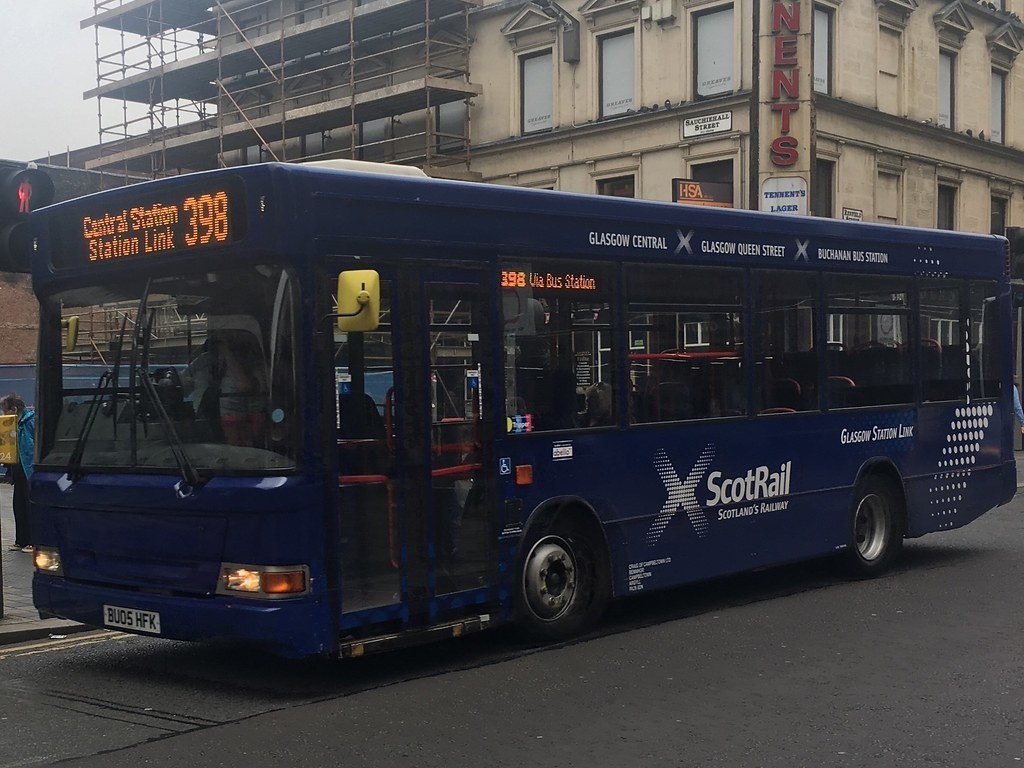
[565,339,944,427]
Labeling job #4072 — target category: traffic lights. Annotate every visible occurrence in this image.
[0,164,56,275]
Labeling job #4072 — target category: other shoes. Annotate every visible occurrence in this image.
[21,544,35,553]
[8,544,21,551]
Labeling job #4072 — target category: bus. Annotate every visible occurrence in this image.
[28,158,1021,663]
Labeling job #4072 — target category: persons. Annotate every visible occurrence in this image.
[0,393,36,553]
[158,311,269,420]
